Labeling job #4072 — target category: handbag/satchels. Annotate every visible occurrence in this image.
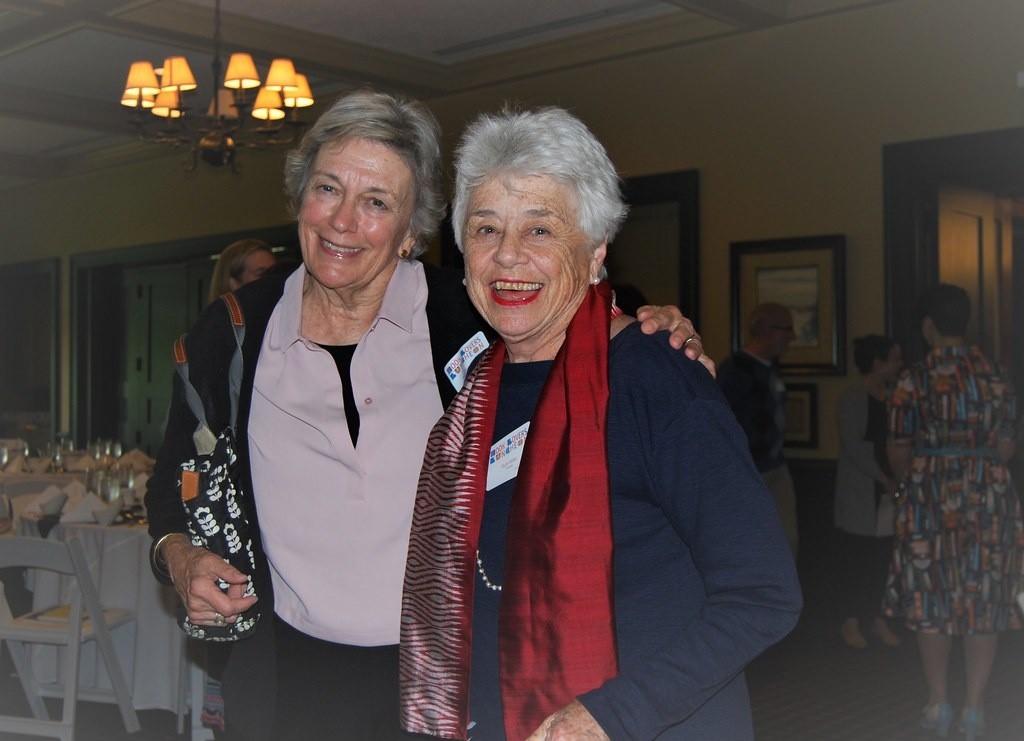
[171,290,263,642]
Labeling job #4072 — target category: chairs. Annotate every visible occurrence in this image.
[0,533,142,741]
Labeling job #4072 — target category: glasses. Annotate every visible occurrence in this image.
[765,324,793,333]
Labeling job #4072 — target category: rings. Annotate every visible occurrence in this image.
[214,613,226,623]
[684,335,703,347]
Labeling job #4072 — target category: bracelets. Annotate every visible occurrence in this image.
[153,532,190,578]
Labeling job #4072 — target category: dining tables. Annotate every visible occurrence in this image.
[0,451,194,712]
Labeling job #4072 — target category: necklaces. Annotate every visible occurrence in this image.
[476,548,502,591]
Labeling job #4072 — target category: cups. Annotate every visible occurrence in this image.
[0,438,134,505]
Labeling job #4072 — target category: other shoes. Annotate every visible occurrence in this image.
[839,620,867,648]
[870,616,902,647]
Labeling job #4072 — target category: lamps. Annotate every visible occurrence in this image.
[121,0,314,167]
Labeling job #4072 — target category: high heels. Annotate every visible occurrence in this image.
[919,701,953,737]
[958,707,986,741]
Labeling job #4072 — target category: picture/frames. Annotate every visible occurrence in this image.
[783,383,820,449]
[603,169,700,337]
[728,233,848,381]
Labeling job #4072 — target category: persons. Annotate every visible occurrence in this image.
[208,238,278,304]
[884,280,1024,740]
[398,109,803,741]
[833,333,902,649]
[716,303,797,560]
[139,78,714,741]
